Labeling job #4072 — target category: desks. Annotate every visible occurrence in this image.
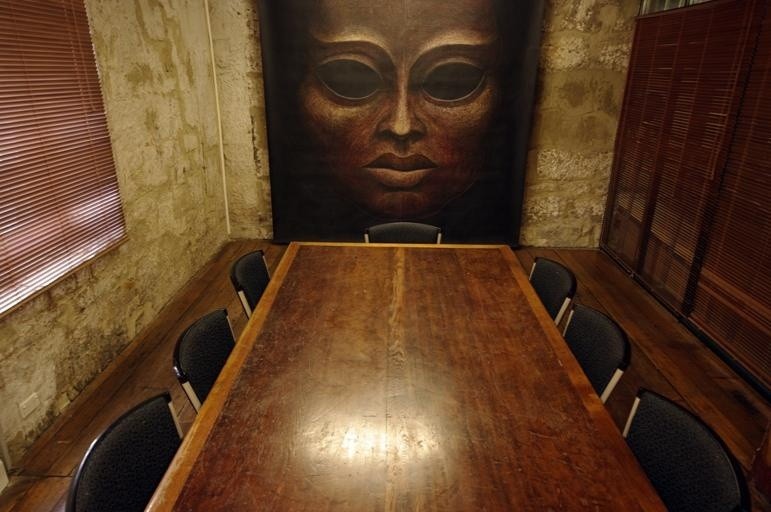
[141,240,667,512]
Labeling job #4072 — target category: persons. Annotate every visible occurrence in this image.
[286,0,512,222]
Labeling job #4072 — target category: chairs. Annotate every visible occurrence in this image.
[227,252,272,319]
[364,221,441,244]
[561,301,636,404]
[67,385,186,512]
[530,256,580,326]
[170,306,240,414]
[619,386,750,512]
